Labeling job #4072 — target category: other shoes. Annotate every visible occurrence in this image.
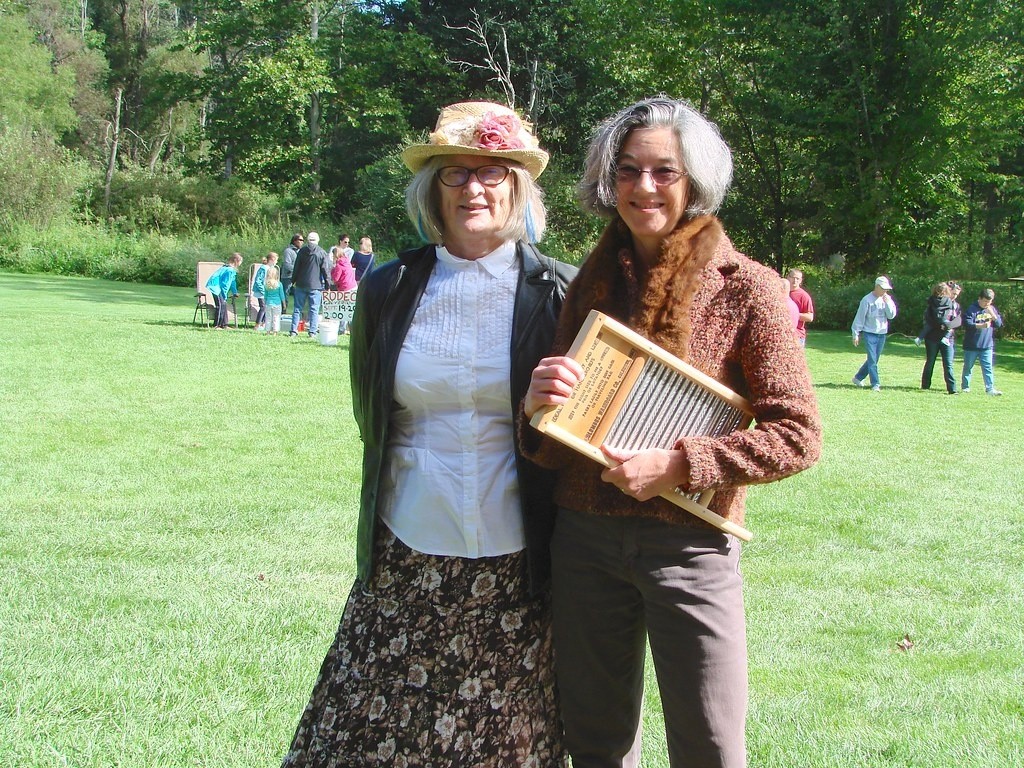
[290,332,296,337]
[273,331,277,335]
[914,337,922,348]
[852,377,864,387]
[254,326,264,332]
[266,331,270,335]
[987,390,1002,396]
[214,325,221,329]
[309,333,314,336]
[223,325,230,329]
[870,384,879,391]
[962,387,969,392]
[949,390,959,395]
[941,337,950,346]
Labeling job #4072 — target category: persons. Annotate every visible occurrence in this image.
[518,97,821,768]
[851,277,897,391]
[780,269,814,345]
[280,102,580,768]
[253,232,376,338]
[206,253,243,330]
[962,289,1004,395]
[914,280,962,396]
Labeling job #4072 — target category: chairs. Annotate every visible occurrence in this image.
[193,261,239,328]
[245,263,280,328]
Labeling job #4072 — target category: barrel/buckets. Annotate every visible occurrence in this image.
[318,321,338,345]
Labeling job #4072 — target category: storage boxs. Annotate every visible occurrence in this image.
[280,314,293,331]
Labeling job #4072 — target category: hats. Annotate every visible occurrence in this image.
[402,102,550,183]
[307,232,319,241]
[875,276,892,290]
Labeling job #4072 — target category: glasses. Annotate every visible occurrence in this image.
[611,164,687,186]
[296,239,304,242]
[344,242,350,244]
[359,243,362,244]
[436,165,512,186]
